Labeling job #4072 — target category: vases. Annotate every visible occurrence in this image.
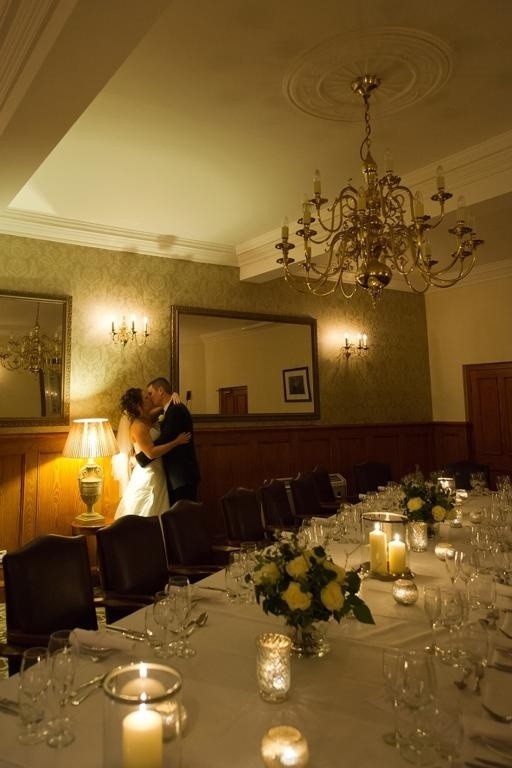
[427,518,440,539]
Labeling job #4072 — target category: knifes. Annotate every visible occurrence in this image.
[105,625,144,642]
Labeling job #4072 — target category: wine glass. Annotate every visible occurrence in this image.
[224,542,257,602]
[444,546,480,588]
[144,575,198,659]
[423,577,507,667]
[467,470,511,551]
[405,468,451,488]
[362,481,407,511]
[381,647,465,768]
[17,631,80,748]
[296,502,360,547]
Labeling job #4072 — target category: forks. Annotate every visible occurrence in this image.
[478,701,512,725]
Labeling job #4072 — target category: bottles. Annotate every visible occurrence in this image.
[450,503,462,528]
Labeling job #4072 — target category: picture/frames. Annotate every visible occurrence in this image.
[283,367,312,402]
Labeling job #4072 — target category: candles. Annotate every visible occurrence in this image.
[388,531,406,573]
[369,519,389,575]
[122,704,164,768]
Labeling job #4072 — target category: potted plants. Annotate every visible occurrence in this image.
[400,466,454,521]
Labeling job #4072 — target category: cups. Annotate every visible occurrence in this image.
[256,632,292,704]
[101,663,183,768]
[410,522,428,552]
[361,512,409,580]
[436,477,455,494]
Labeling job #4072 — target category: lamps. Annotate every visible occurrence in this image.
[275,75,485,307]
[337,333,369,363]
[61,418,120,525]
[0,302,63,380]
[110,315,150,351]
[186,390,192,408]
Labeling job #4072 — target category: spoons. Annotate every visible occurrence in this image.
[454,672,471,689]
[186,613,208,637]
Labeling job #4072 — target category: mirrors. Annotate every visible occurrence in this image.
[0,289,73,426]
[171,304,320,421]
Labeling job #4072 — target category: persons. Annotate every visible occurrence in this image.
[114,388,192,520]
[129,378,202,508]
